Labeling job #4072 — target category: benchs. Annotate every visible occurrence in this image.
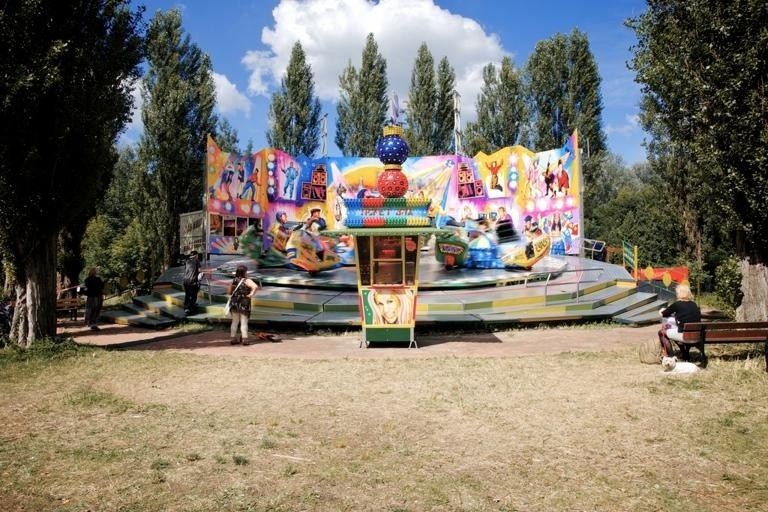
[672,319,767,371]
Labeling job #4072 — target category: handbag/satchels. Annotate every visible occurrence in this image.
[224,298,231,316]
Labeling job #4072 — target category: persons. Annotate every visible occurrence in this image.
[183,251,202,315]
[368,289,414,325]
[226,265,258,346]
[658,285,701,362]
[84,268,105,331]
[57,300,82,321]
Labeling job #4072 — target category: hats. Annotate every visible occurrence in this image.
[188,251,198,259]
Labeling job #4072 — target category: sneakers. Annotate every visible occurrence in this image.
[229,337,249,345]
